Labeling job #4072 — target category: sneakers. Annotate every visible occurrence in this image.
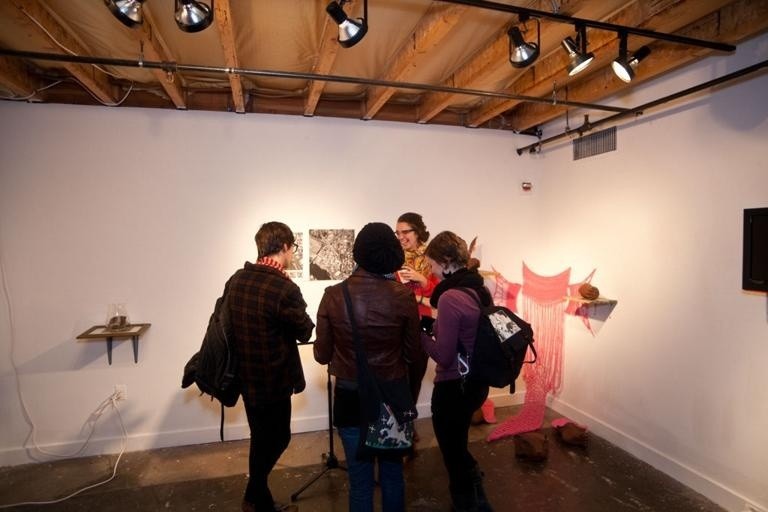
[242,498,298,512]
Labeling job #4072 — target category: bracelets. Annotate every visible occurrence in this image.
[421,297,425,303]
[419,295,424,304]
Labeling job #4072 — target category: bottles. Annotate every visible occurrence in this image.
[106,303,131,331]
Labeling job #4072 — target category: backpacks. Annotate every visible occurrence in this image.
[453,286,538,394]
[181,275,238,408]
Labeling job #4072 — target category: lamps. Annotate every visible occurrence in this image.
[103,0,148,29]
[611,31,652,84]
[506,12,541,68]
[561,22,595,77]
[173,0,214,33]
[325,0,368,47]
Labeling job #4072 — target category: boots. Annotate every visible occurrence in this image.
[447,462,493,512]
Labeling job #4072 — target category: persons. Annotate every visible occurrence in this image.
[222,220,315,512]
[313,221,422,512]
[386,212,442,465]
[419,230,495,512]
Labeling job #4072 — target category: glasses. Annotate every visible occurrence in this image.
[394,228,416,235]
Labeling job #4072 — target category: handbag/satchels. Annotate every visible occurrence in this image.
[355,375,418,465]
